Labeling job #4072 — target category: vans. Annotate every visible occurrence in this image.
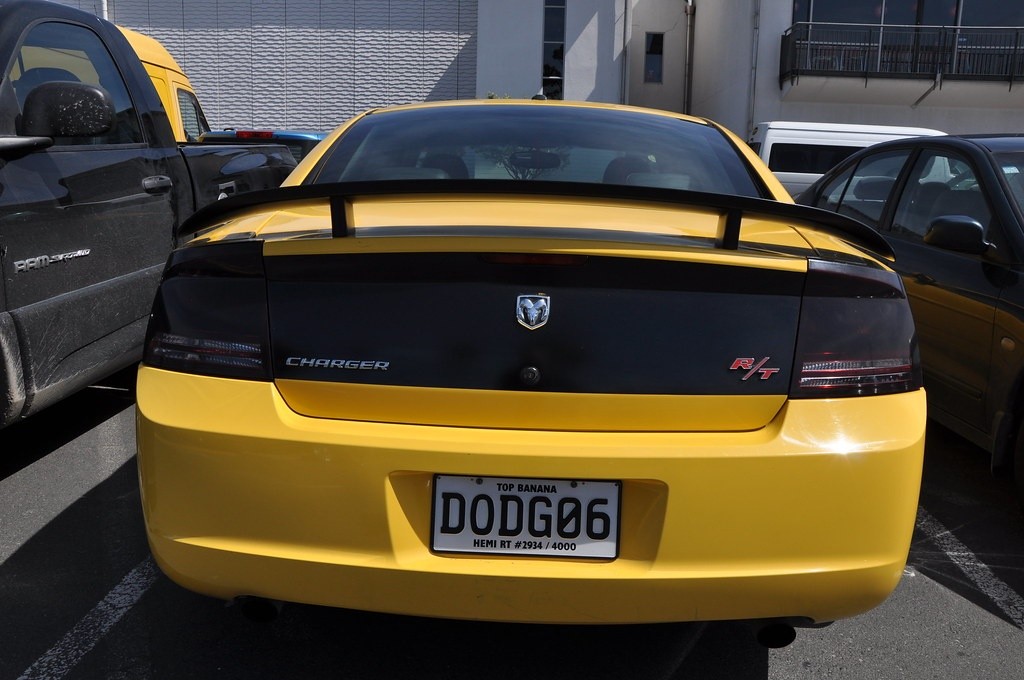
[744,120,1021,202]
[8,21,215,142]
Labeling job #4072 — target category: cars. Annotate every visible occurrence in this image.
[134,96,931,648]
[793,128,1024,508]
[197,126,332,165]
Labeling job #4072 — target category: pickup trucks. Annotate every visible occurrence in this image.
[0,2,301,430]
